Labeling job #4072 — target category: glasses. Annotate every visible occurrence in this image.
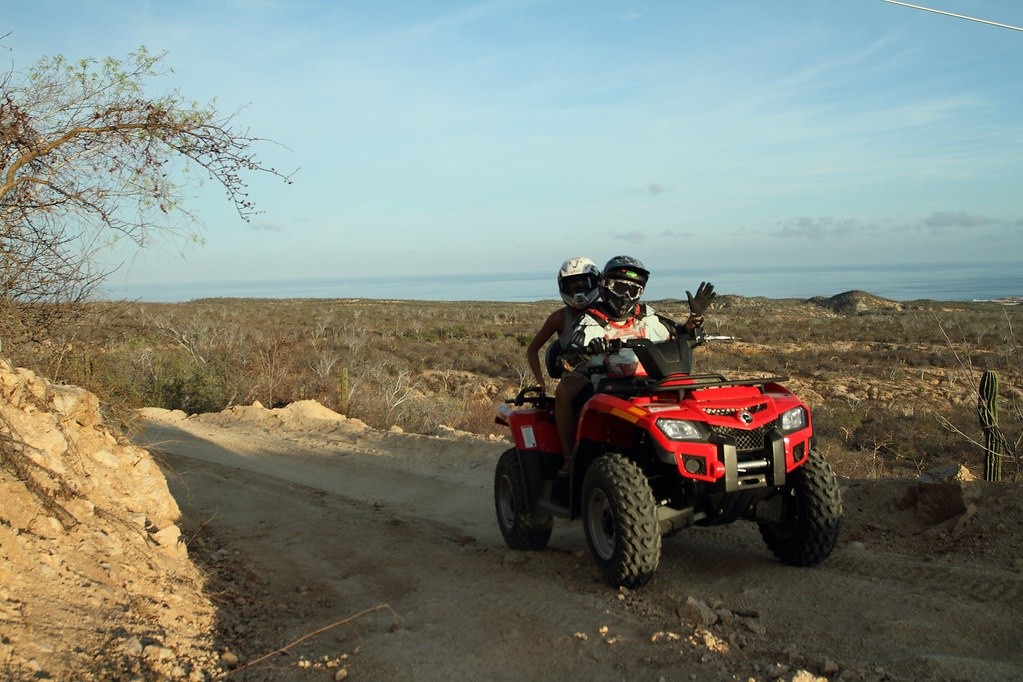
[600,278,643,300]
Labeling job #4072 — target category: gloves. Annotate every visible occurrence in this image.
[686,282,716,315]
[589,337,610,357]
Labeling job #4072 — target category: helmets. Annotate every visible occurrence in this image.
[603,256,650,286]
[557,257,600,311]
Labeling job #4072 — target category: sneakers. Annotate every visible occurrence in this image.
[558,456,571,476]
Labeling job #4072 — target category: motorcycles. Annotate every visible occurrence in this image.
[493,307,844,588]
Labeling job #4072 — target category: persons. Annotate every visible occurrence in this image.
[528,256,603,476]
[560,254,716,420]
